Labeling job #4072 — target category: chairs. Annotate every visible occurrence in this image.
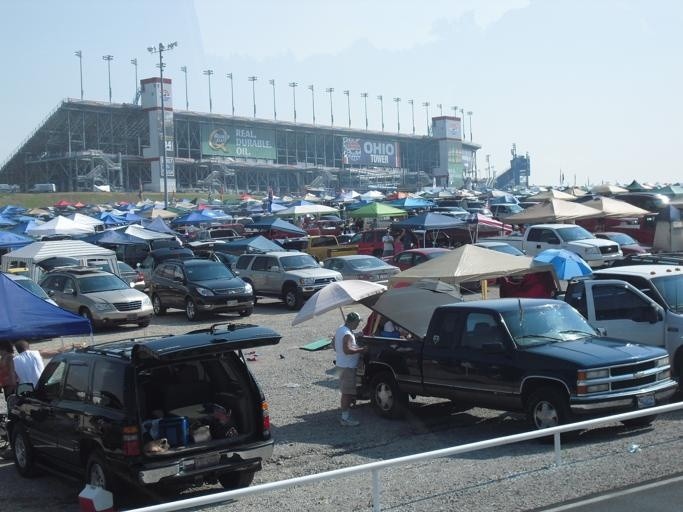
[467,319,497,348]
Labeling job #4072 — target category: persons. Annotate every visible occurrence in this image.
[337,311,366,426]
[12,339,57,392]
[0,342,17,400]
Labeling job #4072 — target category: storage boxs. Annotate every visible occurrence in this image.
[158,415,191,449]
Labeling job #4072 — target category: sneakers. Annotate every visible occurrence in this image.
[338,414,360,428]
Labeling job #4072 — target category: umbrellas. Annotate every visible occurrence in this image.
[536,248,592,280]
[292,276,387,328]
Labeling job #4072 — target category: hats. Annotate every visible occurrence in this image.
[345,312,362,323]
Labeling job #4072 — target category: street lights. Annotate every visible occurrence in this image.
[146,41,181,206]
